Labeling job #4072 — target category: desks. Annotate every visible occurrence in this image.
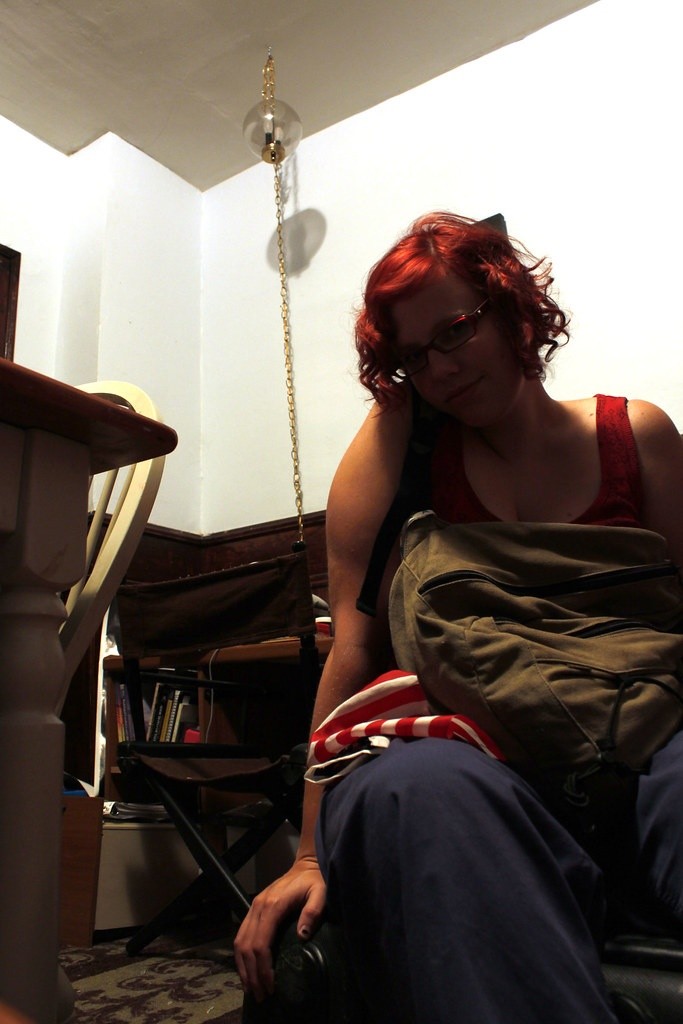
[0,356,178,1024]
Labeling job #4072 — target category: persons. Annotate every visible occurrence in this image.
[235,208,682,1023]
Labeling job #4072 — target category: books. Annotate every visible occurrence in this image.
[115,682,197,743]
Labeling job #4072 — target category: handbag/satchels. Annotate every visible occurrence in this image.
[384,510,683,787]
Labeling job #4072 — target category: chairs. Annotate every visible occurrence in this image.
[112,538,325,960]
[53,380,168,707]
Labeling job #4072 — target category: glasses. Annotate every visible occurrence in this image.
[384,296,493,379]
